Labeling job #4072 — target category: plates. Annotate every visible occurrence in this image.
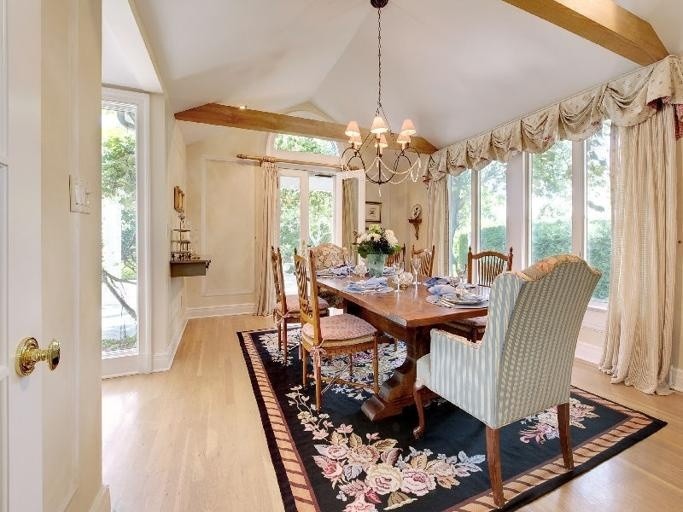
[442,292,489,304]
[410,204,422,219]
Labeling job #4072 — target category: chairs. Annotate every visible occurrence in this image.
[443,247,513,342]
[312,242,347,309]
[386,242,406,269]
[412,255,601,505]
[411,244,436,277]
[293,248,380,413]
[270,246,328,364]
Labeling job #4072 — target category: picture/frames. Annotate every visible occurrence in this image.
[174,186,185,213]
[364,201,382,222]
[68,174,92,214]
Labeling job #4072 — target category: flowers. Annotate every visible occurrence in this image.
[353,224,400,258]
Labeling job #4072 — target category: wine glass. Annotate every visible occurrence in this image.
[344,249,356,277]
[392,263,406,293]
[456,265,467,290]
[411,260,421,287]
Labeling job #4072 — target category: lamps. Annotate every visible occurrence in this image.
[341,1,421,196]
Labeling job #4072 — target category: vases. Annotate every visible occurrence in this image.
[367,254,385,278]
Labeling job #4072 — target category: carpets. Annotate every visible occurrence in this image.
[236,323,668,512]
